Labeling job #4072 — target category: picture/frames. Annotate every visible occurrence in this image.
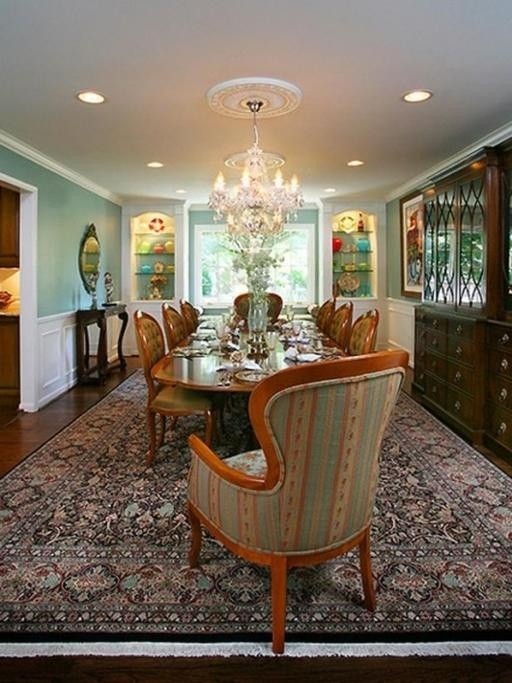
[399,189,422,300]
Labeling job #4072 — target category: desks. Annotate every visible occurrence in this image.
[76,304,129,388]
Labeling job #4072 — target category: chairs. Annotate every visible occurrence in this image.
[182,350,410,656]
[181,299,200,335]
[133,310,227,469]
[345,308,380,357]
[162,302,188,352]
[316,298,335,334]
[234,292,283,313]
[325,301,353,352]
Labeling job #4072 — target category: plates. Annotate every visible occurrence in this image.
[297,352,321,362]
[235,369,274,382]
[338,272,360,292]
[341,216,355,231]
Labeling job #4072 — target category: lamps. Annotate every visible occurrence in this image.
[207,77,303,237]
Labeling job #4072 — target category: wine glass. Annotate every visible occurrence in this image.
[214,321,225,356]
[285,304,303,348]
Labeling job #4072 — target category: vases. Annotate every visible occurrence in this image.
[245,281,269,342]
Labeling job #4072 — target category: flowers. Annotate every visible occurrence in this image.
[231,249,285,316]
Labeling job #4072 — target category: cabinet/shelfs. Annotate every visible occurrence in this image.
[130,212,176,302]
[82,251,99,273]
[411,138,512,466]
[331,208,378,301]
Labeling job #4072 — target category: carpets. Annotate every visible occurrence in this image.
[0,366,512,658]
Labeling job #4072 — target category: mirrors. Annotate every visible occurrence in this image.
[79,223,102,294]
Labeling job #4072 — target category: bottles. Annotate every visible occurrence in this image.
[358,212,364,230]
